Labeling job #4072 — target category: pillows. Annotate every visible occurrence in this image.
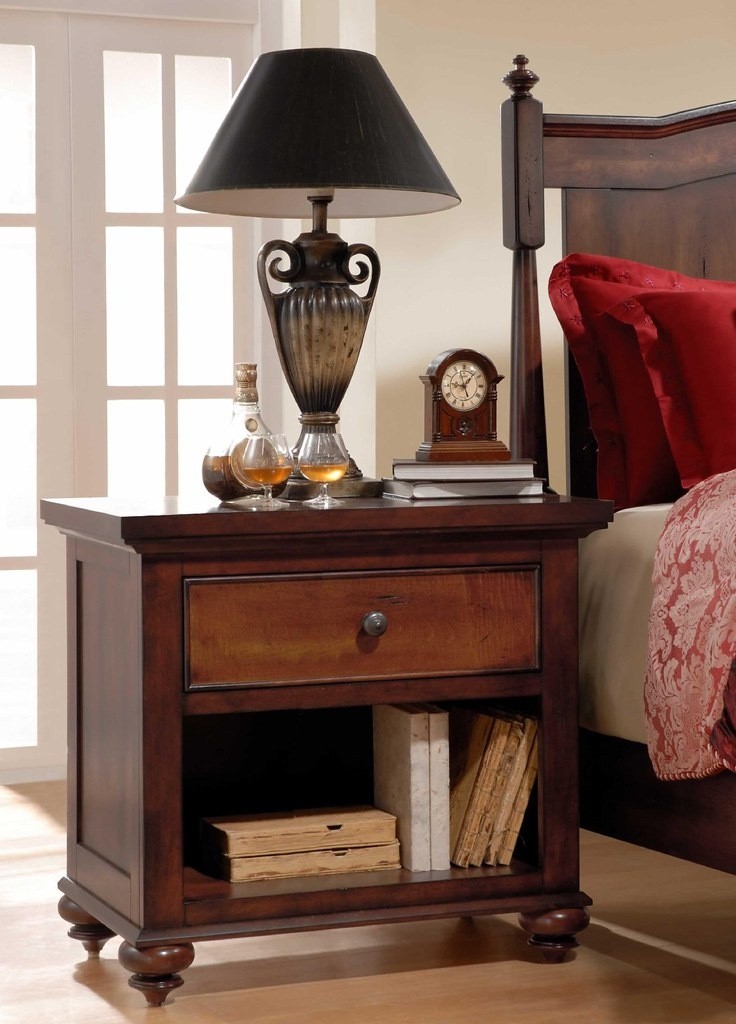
[588,277,736,486]
[547,250,736,510]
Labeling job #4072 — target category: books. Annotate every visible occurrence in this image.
[381,458,546,499]
[201,704,537,884]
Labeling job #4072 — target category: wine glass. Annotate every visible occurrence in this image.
[240,434,290,506]
[298,433,349,508]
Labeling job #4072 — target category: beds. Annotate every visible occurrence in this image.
[500,54,736,875]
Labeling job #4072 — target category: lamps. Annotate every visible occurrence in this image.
[174,49,460,497]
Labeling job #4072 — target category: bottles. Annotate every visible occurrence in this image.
[202,363,287,503]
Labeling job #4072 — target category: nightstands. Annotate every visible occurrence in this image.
[41,490,618,1006]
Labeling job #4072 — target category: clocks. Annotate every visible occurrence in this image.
[418,348,513,465]
